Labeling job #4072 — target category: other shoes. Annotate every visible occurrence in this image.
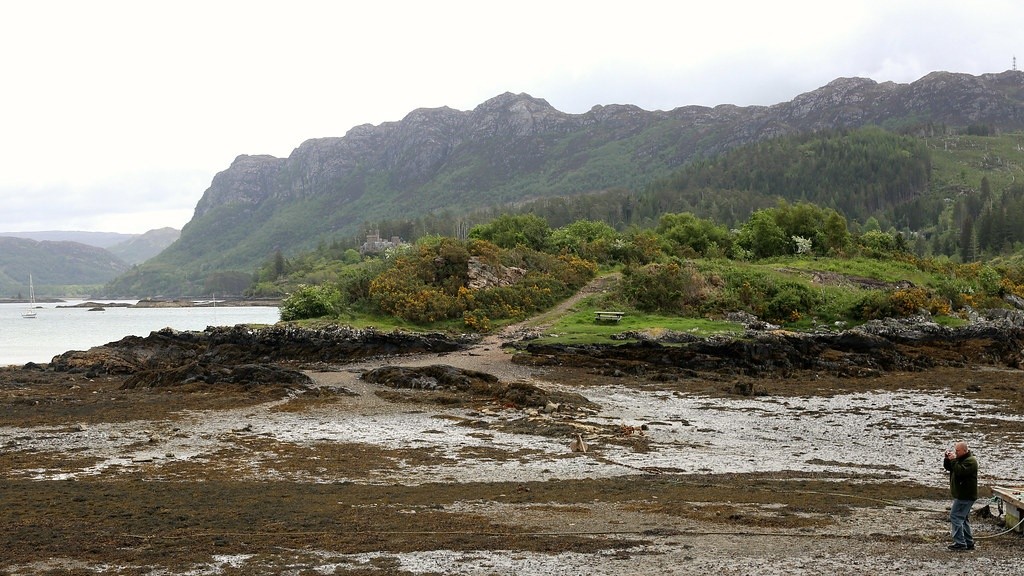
[948,543,968,551]
[966,543,975,550]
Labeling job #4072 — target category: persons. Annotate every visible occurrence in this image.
[943,442,979,551]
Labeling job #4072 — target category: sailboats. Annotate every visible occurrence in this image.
[21,273,39,319]
